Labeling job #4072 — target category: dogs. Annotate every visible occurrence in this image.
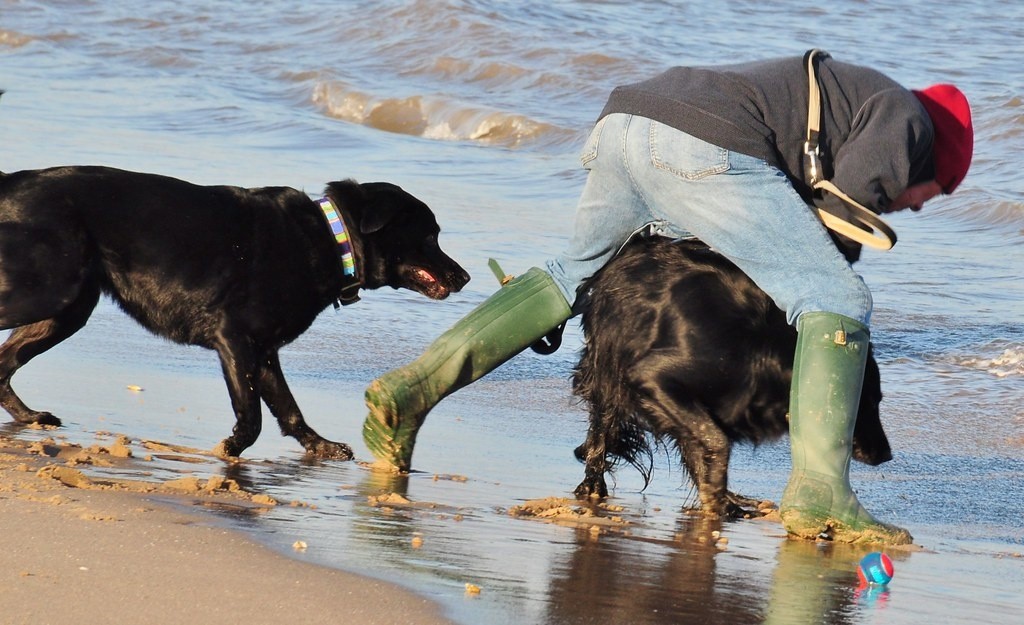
[0,87,471,463]
[569,226,894,523]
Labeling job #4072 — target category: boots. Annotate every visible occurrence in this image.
[361,258,572,471]
[779,311,913,544]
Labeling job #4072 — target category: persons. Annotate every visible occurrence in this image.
[362,46,973,548]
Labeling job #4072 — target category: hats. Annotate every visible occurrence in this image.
[912,84,975,197]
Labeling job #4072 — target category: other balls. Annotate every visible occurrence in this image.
[856,551,895,586]
[854,586,890,607]
